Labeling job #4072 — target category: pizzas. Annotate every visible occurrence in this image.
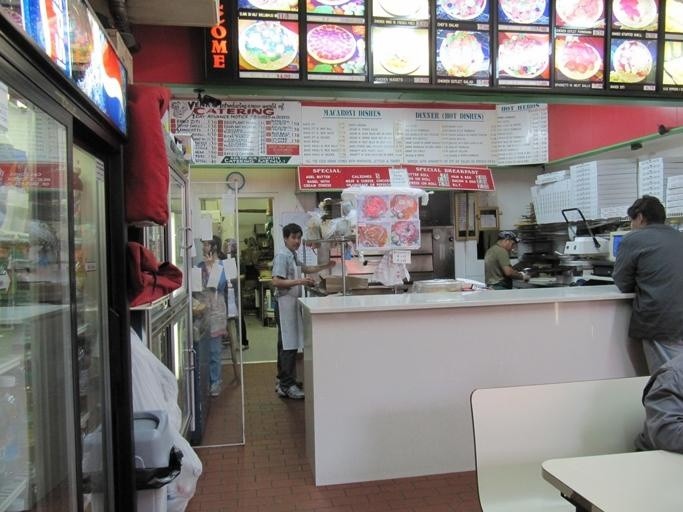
[307,24,356,64]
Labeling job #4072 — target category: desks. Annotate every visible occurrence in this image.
[539,449,681,512]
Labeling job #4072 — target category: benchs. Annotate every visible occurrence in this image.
[468,376,655,512]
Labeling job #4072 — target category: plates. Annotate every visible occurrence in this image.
[556,0,604,27]
[613,0,657,29]
[555,43,601,79]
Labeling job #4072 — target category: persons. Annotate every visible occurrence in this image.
[611,195,683,375]
[197,236,249,397]
[242,237,260,316]
[272,223,336,399]
[484,231,530,290]
[635,353,683,454]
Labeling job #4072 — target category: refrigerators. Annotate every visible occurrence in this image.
[0,0,134,512]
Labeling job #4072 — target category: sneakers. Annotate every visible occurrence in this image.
[274,381,306,401]
[208,381,222,397]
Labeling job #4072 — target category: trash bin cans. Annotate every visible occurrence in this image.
[82,408,168,512]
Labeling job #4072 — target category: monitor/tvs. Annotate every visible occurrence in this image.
[610,231,632,262]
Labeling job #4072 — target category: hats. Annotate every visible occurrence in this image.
[198,235,222,247]
[497,230,521,243]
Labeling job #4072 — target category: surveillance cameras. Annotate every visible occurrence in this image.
[203,95,222,107]
